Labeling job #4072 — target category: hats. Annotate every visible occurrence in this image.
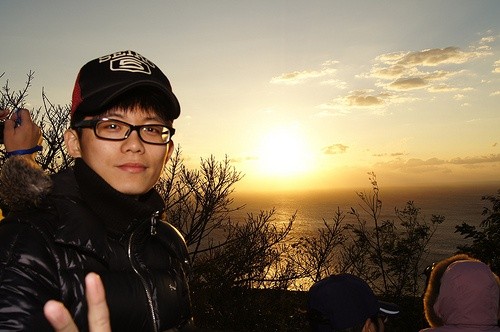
[305,272,399,325]
[70,49,181,121]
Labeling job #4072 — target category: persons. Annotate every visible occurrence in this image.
[0,108,43,221]
[310,273,400,332]
[419,253,500,332]
[0,50,197,332]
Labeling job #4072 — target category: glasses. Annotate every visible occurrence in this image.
[72,117,176,146]
